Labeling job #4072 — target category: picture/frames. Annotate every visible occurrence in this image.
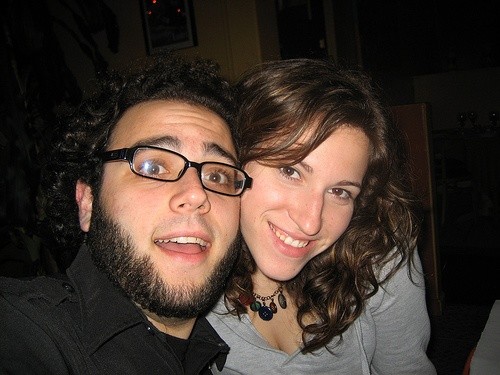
[139,0,198,56]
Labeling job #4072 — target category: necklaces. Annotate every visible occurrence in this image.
[232,281,286,323]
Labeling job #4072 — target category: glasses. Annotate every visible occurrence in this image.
[103,144,252,197]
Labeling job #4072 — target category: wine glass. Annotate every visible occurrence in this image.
[456,112,467,137]
[466,111,481,136]
[488,111,500,134]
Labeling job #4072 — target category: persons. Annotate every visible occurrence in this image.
[204,58,438,375]
[1,53,253,375]
